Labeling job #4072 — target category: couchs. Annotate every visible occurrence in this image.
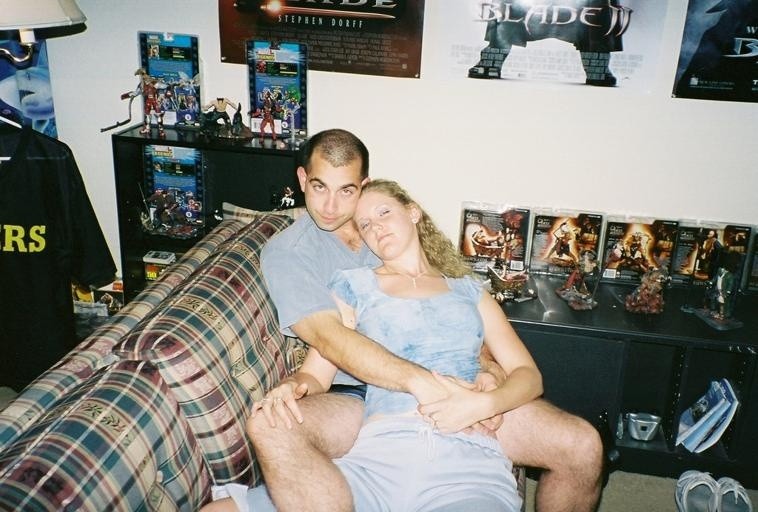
[1,212,310,512]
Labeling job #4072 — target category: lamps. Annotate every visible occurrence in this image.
[1,0,87,68]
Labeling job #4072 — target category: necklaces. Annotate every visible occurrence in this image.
[390,267,431,288]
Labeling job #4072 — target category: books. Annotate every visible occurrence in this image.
[669,369,742,453]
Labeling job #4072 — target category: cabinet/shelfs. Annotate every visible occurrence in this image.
[113,123,306,308]
[480,273,756,489]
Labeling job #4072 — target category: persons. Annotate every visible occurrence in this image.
[137,184,179,231]
[487,226,735,317]
[245,127,604,510]
[199,178,545,511]
[120,67,284,144]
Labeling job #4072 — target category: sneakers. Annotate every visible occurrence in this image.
[676,470,720,512]
[717,477,752,512]
